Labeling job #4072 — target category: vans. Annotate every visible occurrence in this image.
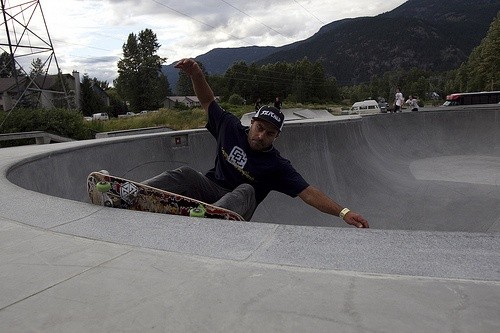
[347,100,381,115]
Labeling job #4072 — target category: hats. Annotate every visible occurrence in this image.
[252,105,285,132]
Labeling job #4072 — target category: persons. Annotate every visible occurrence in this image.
[274,96,283,111]
[379,88,420,113]
[254,98,262,114]
[132,57,371,231]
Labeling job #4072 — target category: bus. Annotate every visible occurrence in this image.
[441,90,500,108]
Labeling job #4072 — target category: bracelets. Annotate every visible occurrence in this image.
[339,207,351,219]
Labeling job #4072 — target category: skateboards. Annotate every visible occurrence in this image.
[86,172,246,222]
